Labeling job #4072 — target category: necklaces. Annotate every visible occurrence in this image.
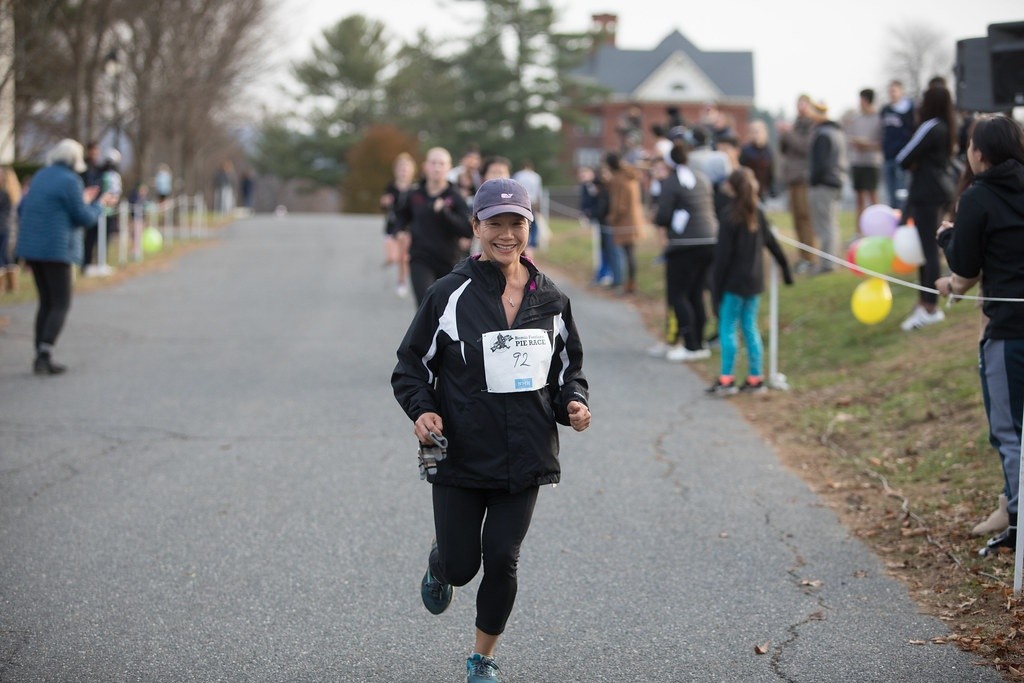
[502,265,521,307]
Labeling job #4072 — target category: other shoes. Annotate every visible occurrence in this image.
[980,525,1017,555]
[738,376,765,396]
[901,305,945,331]
[702,375,738,397]
[33,350,67,376]
[667,345,711,363]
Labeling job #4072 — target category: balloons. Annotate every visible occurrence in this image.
[849,204,924,323]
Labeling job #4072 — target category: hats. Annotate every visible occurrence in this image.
[471,177,533,223]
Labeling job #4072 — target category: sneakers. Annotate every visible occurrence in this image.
[421,537,452,615]
[465,652,500,683]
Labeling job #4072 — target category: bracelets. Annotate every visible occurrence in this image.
[946,279,954,294]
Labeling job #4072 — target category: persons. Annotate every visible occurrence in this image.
[578,102,793,395]
[935,113,1024,548]
[215,161,254,215]
[130,183,146,237]
[848,76,946,219]
[895,87,956,330]
[0,165,24,293]
[156,163,172,224]
[16,139,110,375]
[777,95,846,274]
[80,141,122,275]
[381,147,542,307]
[390,179,591,683]
